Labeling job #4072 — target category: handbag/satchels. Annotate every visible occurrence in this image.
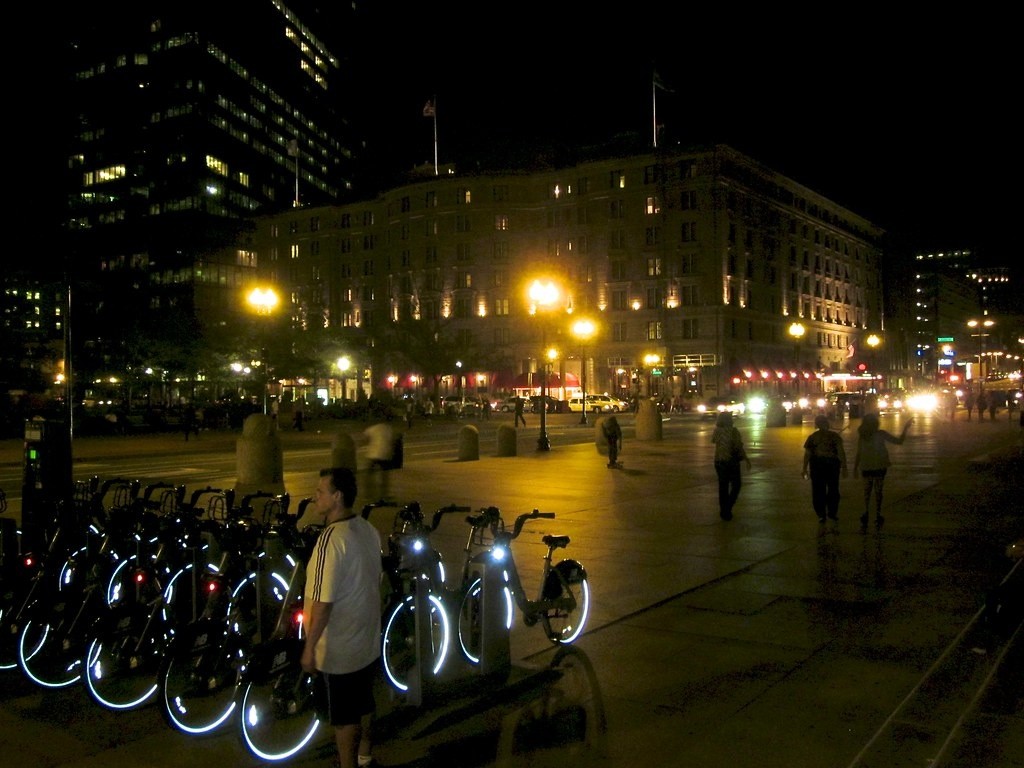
[729,440,745,462]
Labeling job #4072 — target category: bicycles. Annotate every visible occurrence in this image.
[0,473,589,764]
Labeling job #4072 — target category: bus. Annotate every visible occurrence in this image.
[54,374,271,433]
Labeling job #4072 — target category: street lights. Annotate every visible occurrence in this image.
[530,279,561,451]
[867,335,880,393]
[248,287,280,415]
[572,321,594,423]
[788,323,804,408]
[967,317,995,396]
[645,354,659,400]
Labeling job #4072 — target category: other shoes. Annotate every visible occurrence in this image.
[818,515,828,525]
[335,758,380,768]
[827,513,841,521]
[876,515,884,520]
[859,512,869,520]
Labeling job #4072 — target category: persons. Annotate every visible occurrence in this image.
[712,412,751,520]
[364,412,404,500]
[407,396,528,427]
[184,398,307,440]
[631,390,683,415]
[947,387,1024,426]
[301,467,381,768]
[602,411,621,468]
[800,415,848,523]
[853,415,914,523]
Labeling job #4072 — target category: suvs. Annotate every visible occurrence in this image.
[441,396,482,409]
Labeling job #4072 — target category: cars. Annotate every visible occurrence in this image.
[490,394,687,413]
[698,391,865,422]
[877,384,970,412]
